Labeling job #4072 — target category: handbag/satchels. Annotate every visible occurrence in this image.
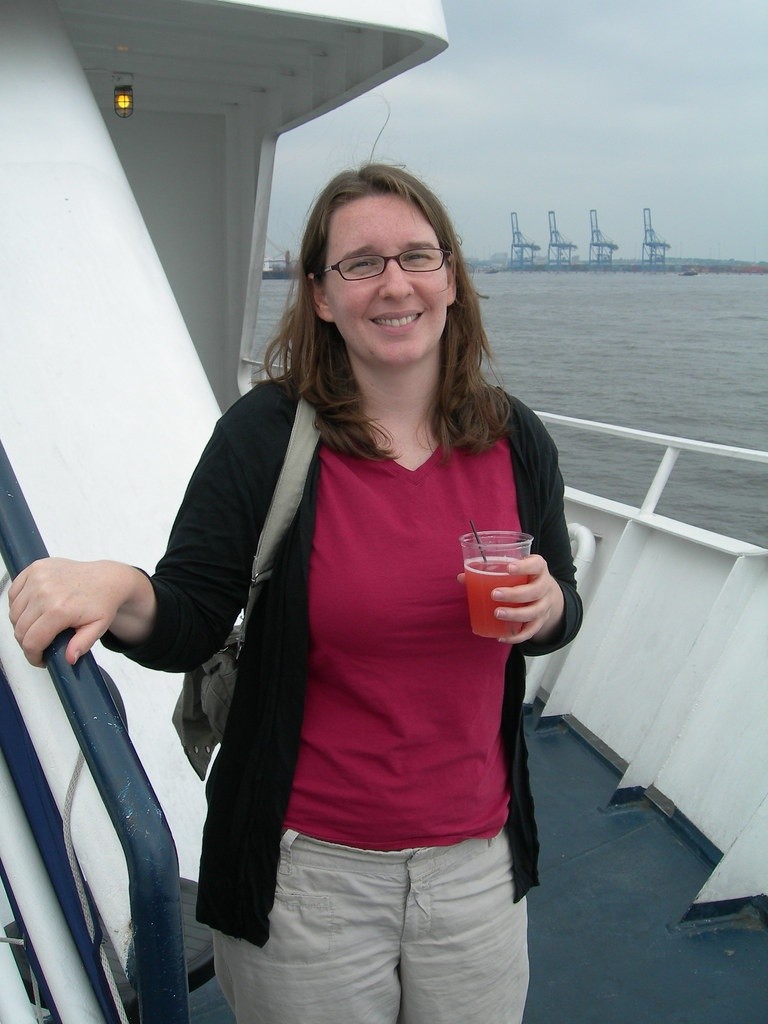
[171,625,246,781]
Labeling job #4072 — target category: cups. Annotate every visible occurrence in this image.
[460,529,533,636]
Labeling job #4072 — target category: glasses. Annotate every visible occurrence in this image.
[324,248,451,281]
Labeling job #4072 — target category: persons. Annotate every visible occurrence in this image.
[7,164,585,1024]
